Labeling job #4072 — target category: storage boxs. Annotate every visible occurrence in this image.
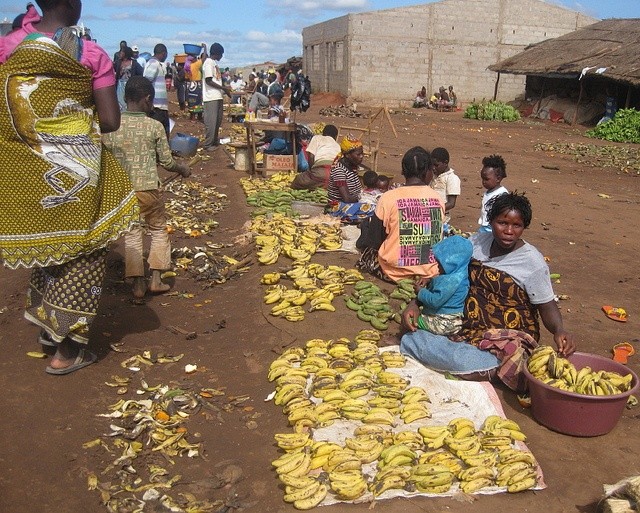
[262,153,294,178]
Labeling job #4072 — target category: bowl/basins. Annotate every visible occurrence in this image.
[357,170,396,190]
[183,43,202,55]
[524,351,640,436]
[173,54,190,63]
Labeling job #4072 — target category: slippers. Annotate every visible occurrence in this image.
[612,341,635,365]
[602,305,628,322]
[46,348,98,376]
[38,333,57,346]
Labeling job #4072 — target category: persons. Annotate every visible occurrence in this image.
[289,124,342,191]
[142,42,169,146]
[322,134,377,224]
[200,54,208,59]
[113,46,144,113]
[402,234,474,336]
[356,169,383,224]
[477,153,510,233]
[414,85,429,109]
[172,53,188,112]
[9,12,31,33]
[0,0,122,376]
[354,145,446,286]
[199,42,233,152]
[432,86,450,110]
[165,74,173,90]
[398,188,577,382]
[131,45,146,70]
[112,40,127,78]
[101,75,193,298]
[428,147,461,236]
[81,33,92,41]
[446,85,457,108]
[430,91,439,106]
[183,41,206,122]
[200,53,208,60]
[171,63,178,90]
[221,63,313,119]
[376,175,390,193]
[165,62,173,91]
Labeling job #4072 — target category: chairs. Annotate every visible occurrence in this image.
[336,105,398,172]
[247,80,258,94]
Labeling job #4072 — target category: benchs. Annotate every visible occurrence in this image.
[438,105,457,112]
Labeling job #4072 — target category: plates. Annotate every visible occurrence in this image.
[220,67,224,73]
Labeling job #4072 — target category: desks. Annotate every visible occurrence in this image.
[243,121,297,178]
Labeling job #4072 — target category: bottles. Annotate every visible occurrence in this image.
[246,109,250,120]
[290,110,295,122]
[278,110,286,123]
[256,106,262,121]
[251,110,255,121]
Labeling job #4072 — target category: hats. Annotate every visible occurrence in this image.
[132,46,139,52]
[269,73,277,80]
[269,105,281,115]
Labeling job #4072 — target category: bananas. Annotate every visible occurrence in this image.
[259,263,418,330]
[549,273,560,278]
[314,121,325,134]
[255,148,263,163]
[583,106,640,144]
[462,99,521,123]
[232,124,247,136]
[248,210,343,265]
[267,329,537,510]
[528,345,633,395]
[229,107,245,116]
[160,271,177,279]
[240,169,329,214]
